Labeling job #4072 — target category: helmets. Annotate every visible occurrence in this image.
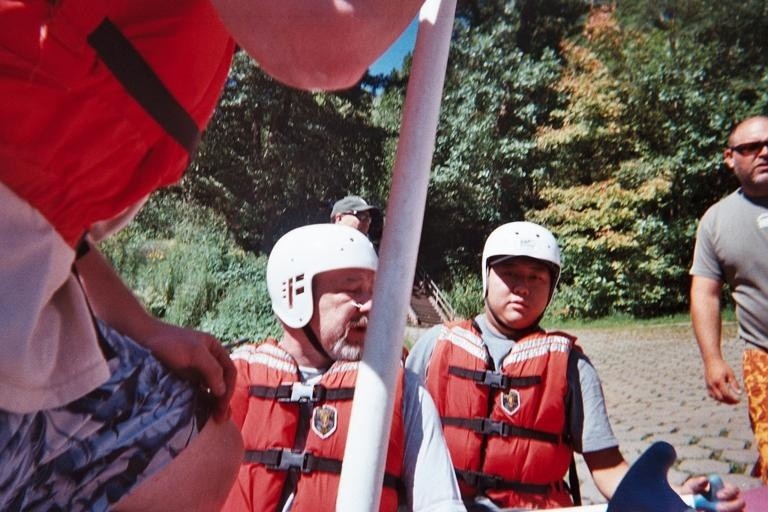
[266,223,379,329]
[481,221,562,297]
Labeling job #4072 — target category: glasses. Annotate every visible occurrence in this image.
[343,212,372,222]
[731,141,768,157]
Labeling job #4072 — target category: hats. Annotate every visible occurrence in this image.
[330,195,374,218]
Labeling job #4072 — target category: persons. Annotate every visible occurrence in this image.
[405,221,747,512]
[330,195,382,237]
[219,224,469,512]
[0,0,426,512]
[688,114,768,486]
